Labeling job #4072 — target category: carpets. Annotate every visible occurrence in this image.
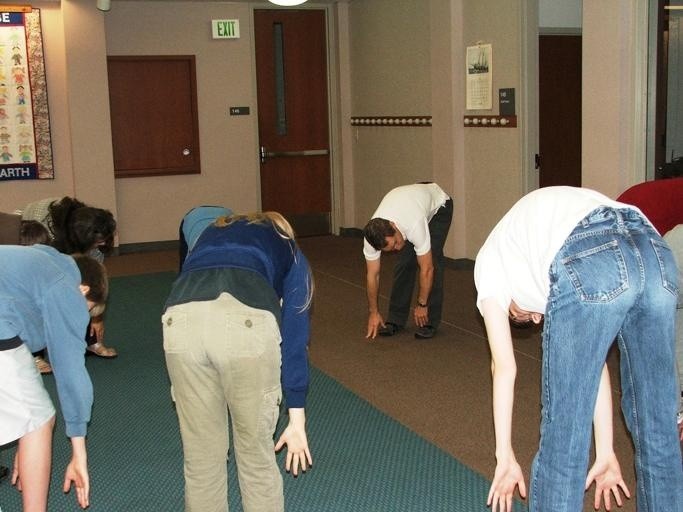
[0,270,527,512]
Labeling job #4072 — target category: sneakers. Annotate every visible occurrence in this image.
[415,325,436,339]
[379,323,403,336]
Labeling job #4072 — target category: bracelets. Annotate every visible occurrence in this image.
[415,300,429,310]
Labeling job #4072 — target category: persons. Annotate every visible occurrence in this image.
[178,204,234,271]
[10,193,119,375]
[361,178,455,342]
[0,211,53,374]
[615,156,683,444]
[0,241,110,512]
[473,183,683,512]
[159,208,318,512]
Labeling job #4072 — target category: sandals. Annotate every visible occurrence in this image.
[86,343,118,359]
[34,356,52,375]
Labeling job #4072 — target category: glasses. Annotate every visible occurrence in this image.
[509,309,544,323]
